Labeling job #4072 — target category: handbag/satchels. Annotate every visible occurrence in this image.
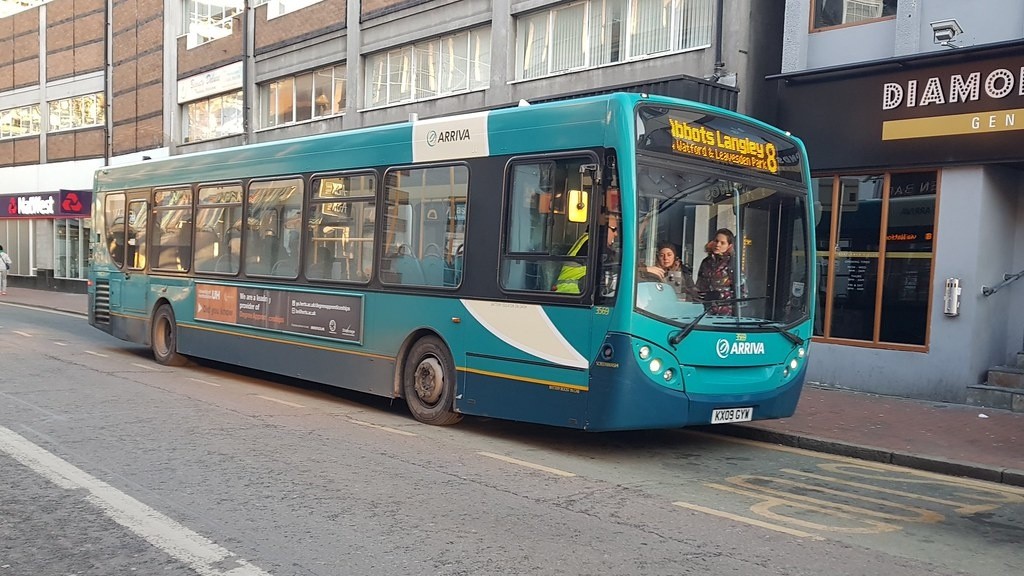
[6,264,9,269]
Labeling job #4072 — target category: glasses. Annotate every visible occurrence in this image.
[608,226,617,232]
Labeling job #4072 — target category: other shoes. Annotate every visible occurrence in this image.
[0,291,6,295]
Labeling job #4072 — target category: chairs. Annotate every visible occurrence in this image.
[110,215,464,291]
[536,261,560,290]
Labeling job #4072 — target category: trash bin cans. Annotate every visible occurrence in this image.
[36,268,54,290]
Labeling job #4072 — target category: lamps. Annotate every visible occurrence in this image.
[931,19,964,49]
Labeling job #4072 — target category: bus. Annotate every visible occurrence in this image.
[81,90,813,454]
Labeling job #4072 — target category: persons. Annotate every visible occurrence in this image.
[553,212,665,295]
[653,242,701,303]
[694,227,748,317]
[0,244,12,295]
